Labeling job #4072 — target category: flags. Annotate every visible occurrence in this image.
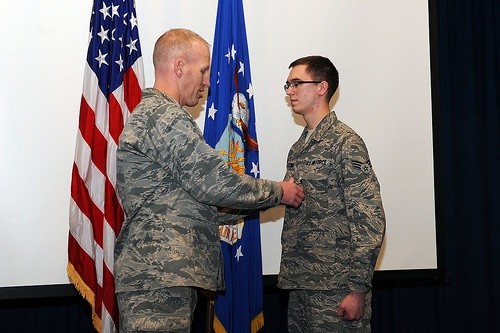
[195,1,269,333]
[63,1,146,333]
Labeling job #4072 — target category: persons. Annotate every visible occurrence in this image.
[276,55,387,333]
[112,28,304,333]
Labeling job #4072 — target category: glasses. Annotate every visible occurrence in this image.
[284,81,320,91]
